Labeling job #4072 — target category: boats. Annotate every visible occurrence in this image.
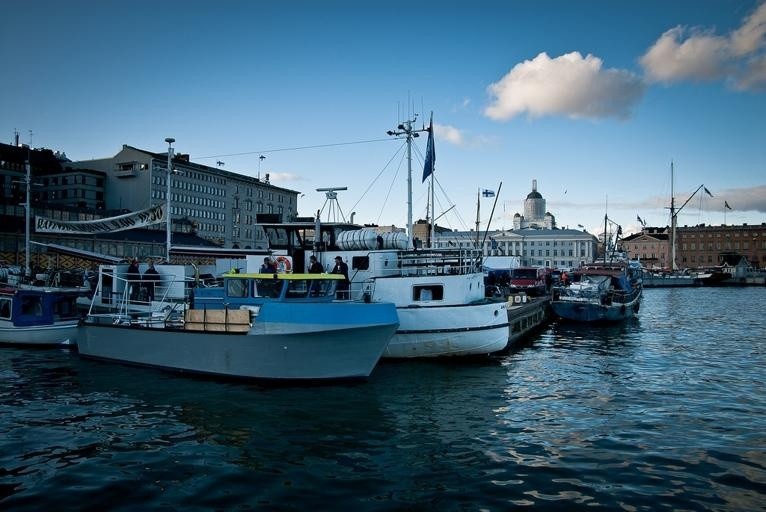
[0,264,93,345]
[76,267,401,379]
[549,213,644,323]
[76,88,511,360]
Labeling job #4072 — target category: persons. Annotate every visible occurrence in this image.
[307,255,324,296]
[261,258,277,298]
[447,267,458,274]
[662,268,666,278]
[562,271,569,285]
[545,270,552,292]
[142,262,161,304]
[128,261,141,304]
[331,256,350,300]
[484,271,511,297]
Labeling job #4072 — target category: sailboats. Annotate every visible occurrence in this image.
[643,183,732,288]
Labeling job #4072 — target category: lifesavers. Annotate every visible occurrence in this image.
[273,257,291,274]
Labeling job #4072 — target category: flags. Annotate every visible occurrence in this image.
[421,120,435,183]
[637,216,644,225]
[725,201,731,209]
[482,188,494,198]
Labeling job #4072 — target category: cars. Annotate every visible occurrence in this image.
[509,267,552,296]
